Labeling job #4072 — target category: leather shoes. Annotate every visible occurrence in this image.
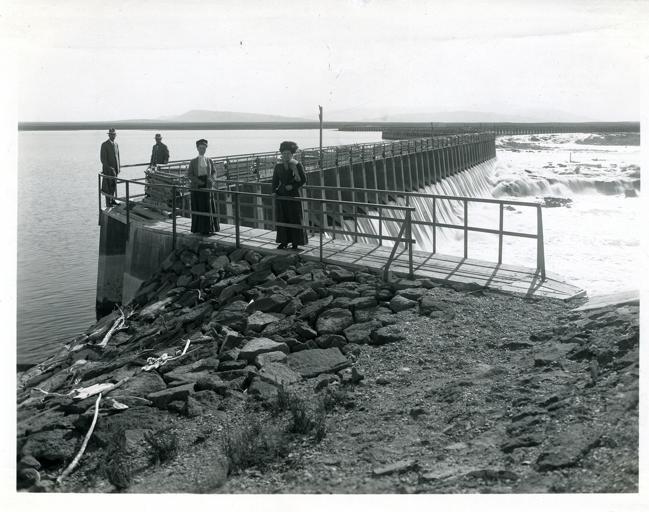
[277,243,287,249]
[106,201,119,207]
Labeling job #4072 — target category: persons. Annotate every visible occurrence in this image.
[100,127,124,208]
[272,141,310,250]
[149,131,170,166]
[187,139,221,237]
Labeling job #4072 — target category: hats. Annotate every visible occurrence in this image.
[107,128,116,134]
[154,134,162,139]
[197,139,207,147]
[280,142,297,154]
